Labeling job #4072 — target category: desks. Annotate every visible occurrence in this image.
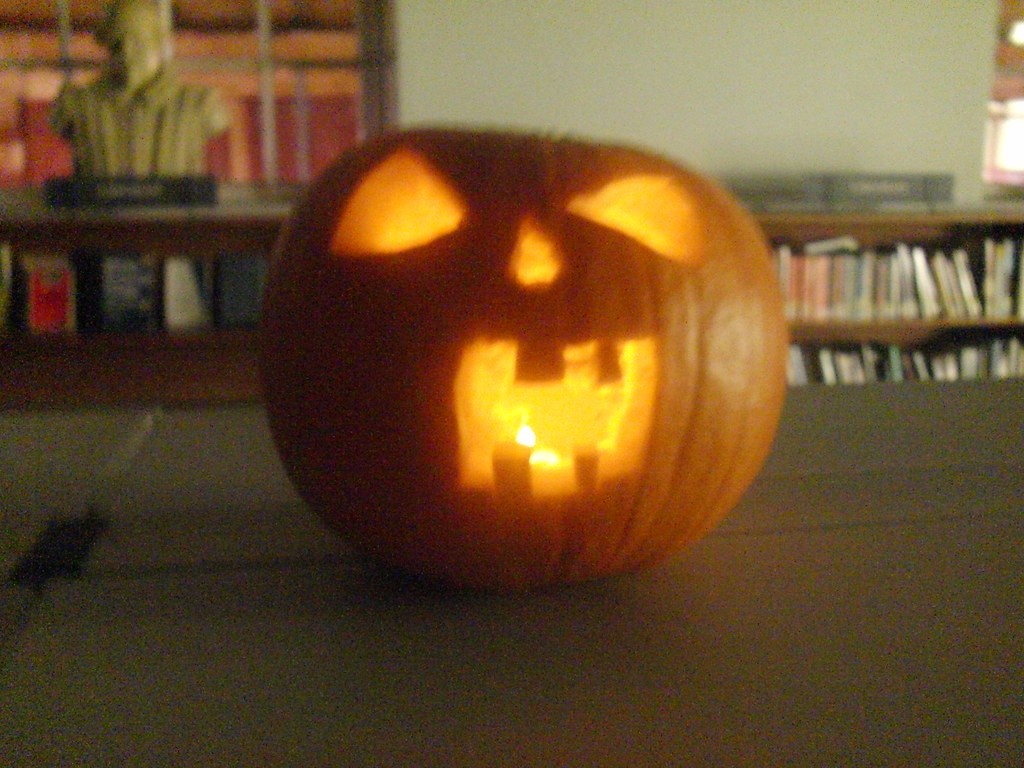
[0,375,1024,768]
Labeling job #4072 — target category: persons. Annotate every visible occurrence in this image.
[51,0,230,177]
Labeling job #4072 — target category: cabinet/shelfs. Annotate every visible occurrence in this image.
[0,181,305,410]
[755,208,1024,346]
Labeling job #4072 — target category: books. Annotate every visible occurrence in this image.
[773,239,1024,389]
[2,241,273,341]
